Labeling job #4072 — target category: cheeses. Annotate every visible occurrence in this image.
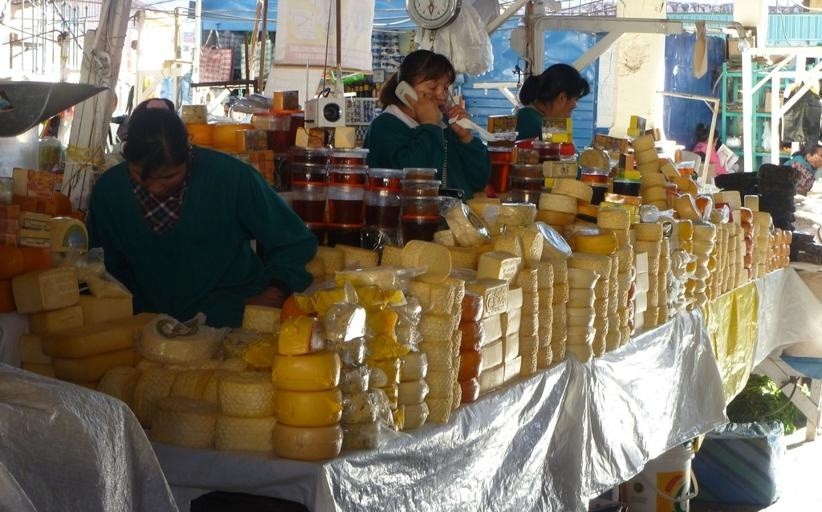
[1,115,794,461]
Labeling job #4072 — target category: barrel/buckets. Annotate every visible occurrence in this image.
[629,443,698,512]
[185,123,215,148]
[214,124,253,152]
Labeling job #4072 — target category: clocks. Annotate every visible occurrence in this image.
[405,0,461,29]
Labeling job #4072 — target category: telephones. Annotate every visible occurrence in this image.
[395,80,443,122]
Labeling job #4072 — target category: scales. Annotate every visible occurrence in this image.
[406,0,462,52]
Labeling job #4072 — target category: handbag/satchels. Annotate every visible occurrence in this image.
[200,31,232,82]
[241,24,271,79]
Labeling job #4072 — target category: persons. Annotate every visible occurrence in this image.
[692,124,727,175]
[85,98,318,327]
[782,143,822,196]
[514,64,589,140]
[363,50,492,200]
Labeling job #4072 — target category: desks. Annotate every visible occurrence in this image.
[113,262,801,510]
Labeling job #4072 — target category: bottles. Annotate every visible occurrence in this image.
[345,80,370,97]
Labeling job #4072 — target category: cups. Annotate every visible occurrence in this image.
[486,132,572,206]
[583,173,641,206]
[291,145,442,248]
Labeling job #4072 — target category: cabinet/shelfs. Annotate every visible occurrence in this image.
[719,63,816,170]
